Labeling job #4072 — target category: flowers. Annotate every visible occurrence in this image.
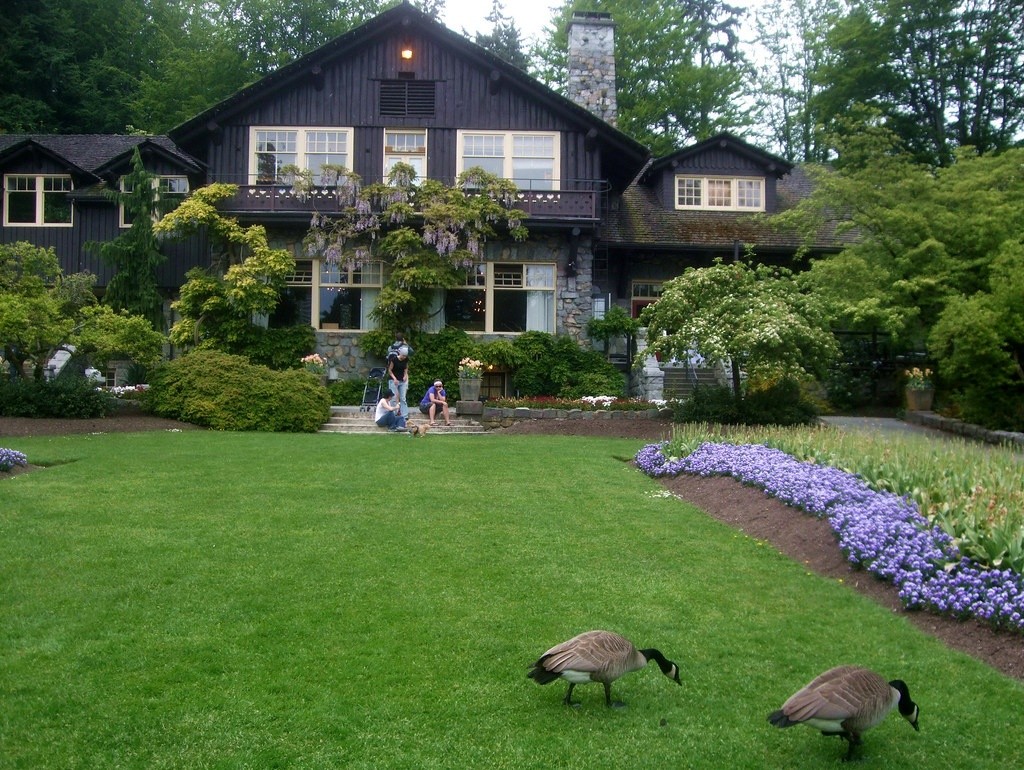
[905,367,935,390]
[457,357,485,381]
[301,353,327,374]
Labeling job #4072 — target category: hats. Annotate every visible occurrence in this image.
[400,347,408,356]
[382,388,395,398]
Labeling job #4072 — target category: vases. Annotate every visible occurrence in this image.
[906,391,934,411]
[459,380,482,401]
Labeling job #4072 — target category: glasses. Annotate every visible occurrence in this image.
[435,386,442,388]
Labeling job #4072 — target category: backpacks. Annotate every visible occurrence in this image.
[387,343,404,366]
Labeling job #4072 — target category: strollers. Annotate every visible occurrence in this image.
[359,366,387,412]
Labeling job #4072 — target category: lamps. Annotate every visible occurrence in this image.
[402,35,413,61]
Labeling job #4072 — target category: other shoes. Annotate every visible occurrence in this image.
[429,422,438,426]
[445,421,452,425]
[395,426,407,432]
[388,428,394,432]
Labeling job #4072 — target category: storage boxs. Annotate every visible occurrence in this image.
[322,323,339,329]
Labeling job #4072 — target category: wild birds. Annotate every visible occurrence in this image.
[525,629,683,710]
[765,665,921,750]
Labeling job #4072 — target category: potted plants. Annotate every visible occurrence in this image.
[85,352,107,381]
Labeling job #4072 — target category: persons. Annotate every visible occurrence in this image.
[419,379,451,425]
[375,389,410,432]
[387,332,414,422]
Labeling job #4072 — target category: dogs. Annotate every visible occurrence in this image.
[405,421,431,438]
